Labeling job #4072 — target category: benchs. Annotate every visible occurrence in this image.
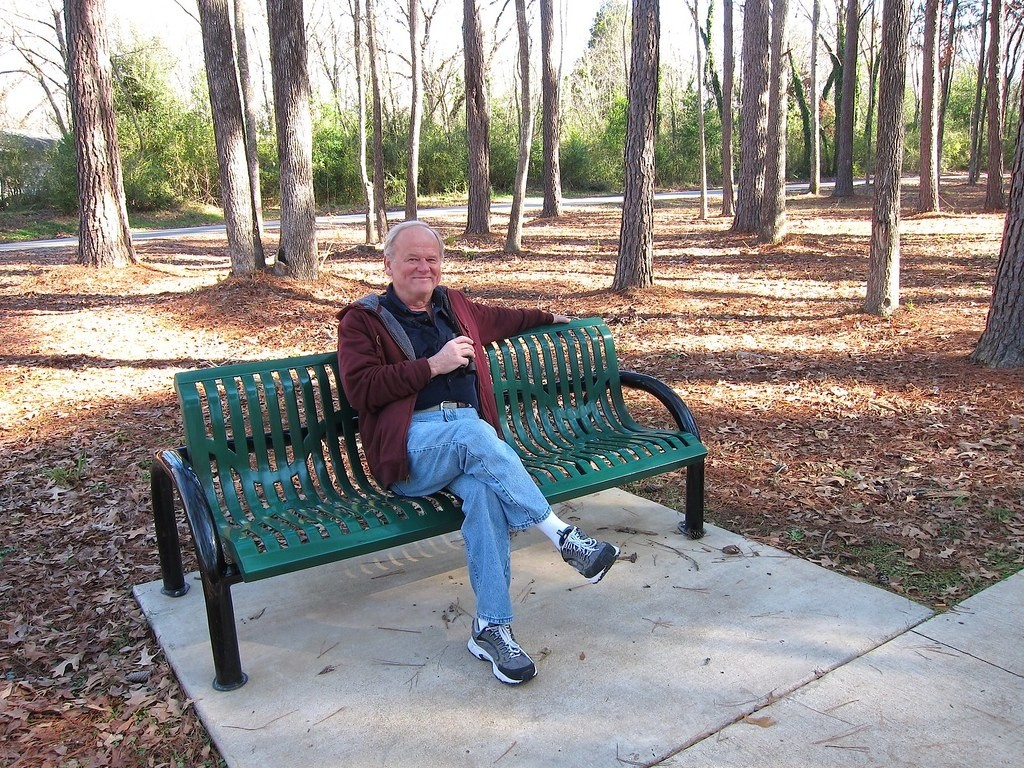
[149,318,708,692]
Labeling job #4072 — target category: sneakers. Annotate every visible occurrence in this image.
[467,618,538,684]
[556,526,621,584]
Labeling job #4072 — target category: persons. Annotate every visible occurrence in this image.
[334,220,619,685]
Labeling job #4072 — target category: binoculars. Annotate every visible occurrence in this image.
[441,332,477,378]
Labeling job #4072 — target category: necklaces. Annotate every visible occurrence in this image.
[411,303,432,309]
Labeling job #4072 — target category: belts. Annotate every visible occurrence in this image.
[411,401,473,412]
